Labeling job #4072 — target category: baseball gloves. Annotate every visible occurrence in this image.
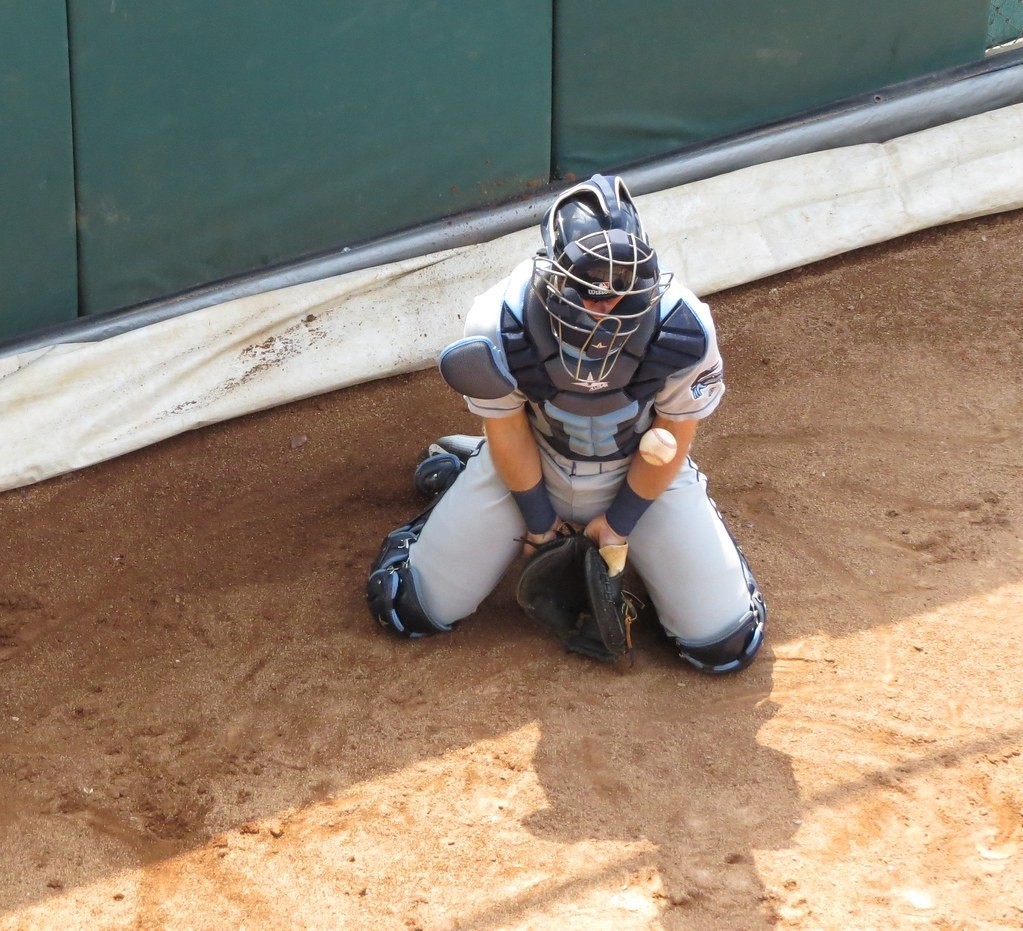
[514,536,643,674]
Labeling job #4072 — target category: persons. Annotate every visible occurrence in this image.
[367,165,767,674]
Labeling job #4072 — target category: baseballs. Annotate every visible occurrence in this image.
[638,428,678,467]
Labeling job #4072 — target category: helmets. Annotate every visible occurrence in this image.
[531,176,673,385]
